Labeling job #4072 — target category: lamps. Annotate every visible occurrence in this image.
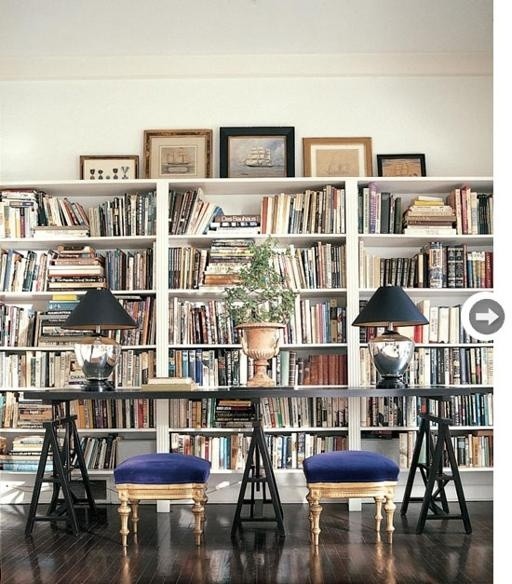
[350,284,431,390]
[57,286,138,394]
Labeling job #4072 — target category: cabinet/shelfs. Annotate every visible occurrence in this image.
[165,176,356,514]
[355,176,492,515]
[0,179,165,512]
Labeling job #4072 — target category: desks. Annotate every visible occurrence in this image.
[20,385,493,535]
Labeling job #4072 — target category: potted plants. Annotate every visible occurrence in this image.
[217,234,300,388]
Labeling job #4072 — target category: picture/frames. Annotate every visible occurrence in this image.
[219,127,295,179]
[303,136,373,177]
[79,154,140,181]
[143,128,213,179]
[376,154,427,176]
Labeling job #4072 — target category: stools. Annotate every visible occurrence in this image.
[112,452,210,557]
[297,450,400,547]
